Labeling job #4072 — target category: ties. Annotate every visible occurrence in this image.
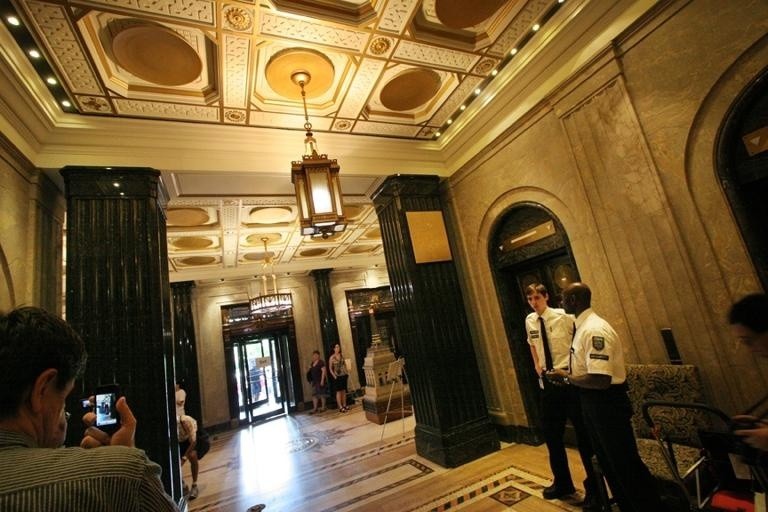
[570,322,576,374]
[538,317,553,370]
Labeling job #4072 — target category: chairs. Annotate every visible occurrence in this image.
[591,365,716,512]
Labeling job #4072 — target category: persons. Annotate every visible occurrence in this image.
[726,293,768,452]
[329,343,352,413]
[175,415,209,497]
[176,382,187,416]
[524,283,596,509]
[249,360,262,400]
[545,282,654,512]
[308,351,326,414]
[1,306,180,510]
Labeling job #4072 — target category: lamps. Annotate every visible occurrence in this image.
[245,232,294,321]
[264,46,348,240]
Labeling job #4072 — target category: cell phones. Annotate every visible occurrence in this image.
[94,384,121,435]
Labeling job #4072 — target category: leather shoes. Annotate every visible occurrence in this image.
[543,484,576,499]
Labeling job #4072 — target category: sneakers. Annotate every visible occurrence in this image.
[183,485,198,497]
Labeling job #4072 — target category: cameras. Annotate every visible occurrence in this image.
[80,398,93,410]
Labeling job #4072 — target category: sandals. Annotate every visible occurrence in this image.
[338,406,351,412]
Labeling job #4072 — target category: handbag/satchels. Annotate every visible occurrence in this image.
[307,368,313,381]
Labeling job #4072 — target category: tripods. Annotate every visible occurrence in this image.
[377,372,414,455]
